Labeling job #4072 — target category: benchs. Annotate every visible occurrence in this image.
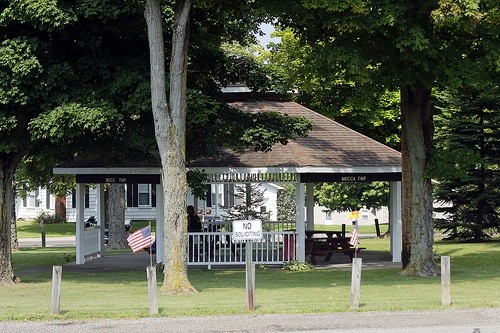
[305,247,366,254]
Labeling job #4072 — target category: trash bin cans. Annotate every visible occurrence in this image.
[282,229,297,262]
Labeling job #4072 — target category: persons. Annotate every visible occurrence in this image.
[187,205,203,237]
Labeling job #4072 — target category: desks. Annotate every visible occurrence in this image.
[305,229,355,264]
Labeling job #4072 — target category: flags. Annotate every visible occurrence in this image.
[127,225,153,255]
[350,220,358,250]
